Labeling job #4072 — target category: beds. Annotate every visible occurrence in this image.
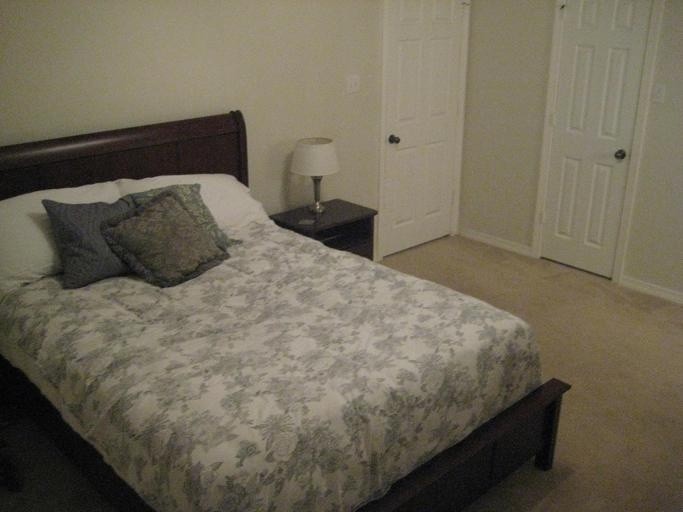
[0,110,569,512]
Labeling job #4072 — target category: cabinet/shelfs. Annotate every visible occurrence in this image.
[267,198,377,260]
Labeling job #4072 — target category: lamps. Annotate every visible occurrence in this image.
[289,136,342,214]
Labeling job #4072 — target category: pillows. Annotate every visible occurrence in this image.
[0,172,265,288]
